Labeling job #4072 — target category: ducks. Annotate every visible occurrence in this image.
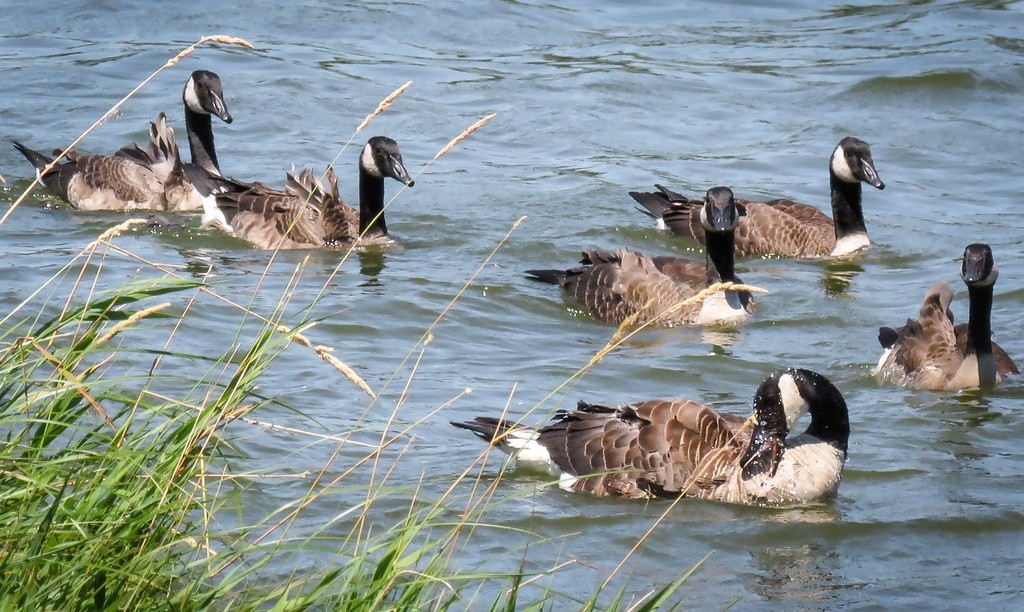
[448,367,851,510]
[628,136,887,263]
[868,243,1021,393]
[181,136,415,251]
[12,69,234,212]
[521,186,755,330]
